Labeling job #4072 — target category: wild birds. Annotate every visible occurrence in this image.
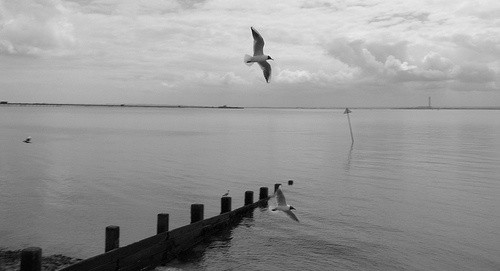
[243,26,274,84]
[23,138,32,143]
[270,184,301,223]
[222,189,231,197]
